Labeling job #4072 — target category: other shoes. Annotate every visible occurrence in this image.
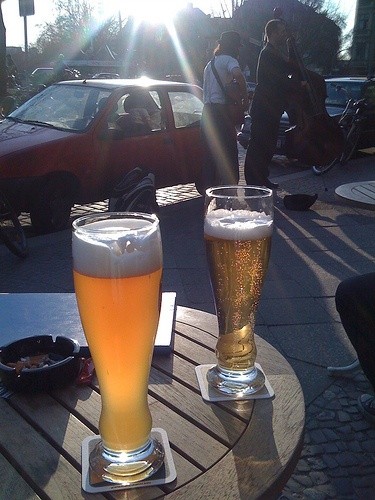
[248,180,278,188]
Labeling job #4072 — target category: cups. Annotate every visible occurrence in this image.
[72,210,167,487]
[203,186,273,398]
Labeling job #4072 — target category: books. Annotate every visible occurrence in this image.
[153,292,177,353]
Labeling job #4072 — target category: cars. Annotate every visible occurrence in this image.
[1,73,240,234]
[245,81,256,96]
[237,77,375,163]
[165,74,200,84]
[32,67,79,79]
[92,73,120,79]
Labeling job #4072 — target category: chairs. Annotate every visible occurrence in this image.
[117,95,148,125]
[97,97,120,123]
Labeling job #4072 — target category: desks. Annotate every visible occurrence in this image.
[0,305,306,500]
[335,181,375,210]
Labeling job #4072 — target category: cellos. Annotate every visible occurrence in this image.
[272,7,343,192]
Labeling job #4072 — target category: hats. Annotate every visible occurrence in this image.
[219,31,244,47]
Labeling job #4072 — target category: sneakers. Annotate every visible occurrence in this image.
[357,394,375,424]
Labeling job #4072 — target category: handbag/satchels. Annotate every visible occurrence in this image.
[210,56,242,122]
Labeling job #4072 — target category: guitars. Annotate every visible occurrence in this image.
[226,77,245,124]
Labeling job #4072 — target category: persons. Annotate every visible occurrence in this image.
[201,31,250,211]
[53,54,73,82]
[244,18,311,200]
[6,54,17,72]
